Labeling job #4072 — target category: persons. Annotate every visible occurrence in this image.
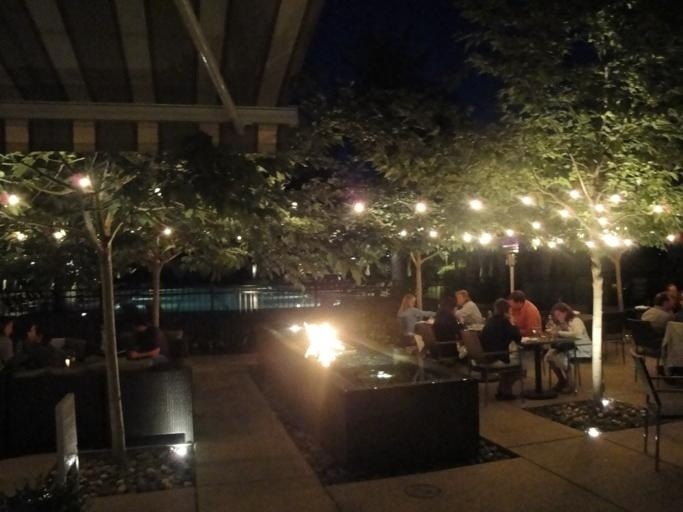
[23,322,46,352]
[642,284,683,387]
[398,289,593,401]
[127,318,170,359]
[1,318,17,367]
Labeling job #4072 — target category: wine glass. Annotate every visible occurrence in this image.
[531,319,541,338]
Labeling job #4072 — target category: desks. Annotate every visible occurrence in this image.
[462,324,485,371]
[518,336,566,400]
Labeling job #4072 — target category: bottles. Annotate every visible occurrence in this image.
[545,314,558,334]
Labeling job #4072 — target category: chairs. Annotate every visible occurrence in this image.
[461,329,525,408]
[414,322,461,382]
[634,305,650,320]
[627,319,664,387]
[386,323,418,356]
[0,322,198,466]
[548,319,607,394]
[629,347,683,473]
